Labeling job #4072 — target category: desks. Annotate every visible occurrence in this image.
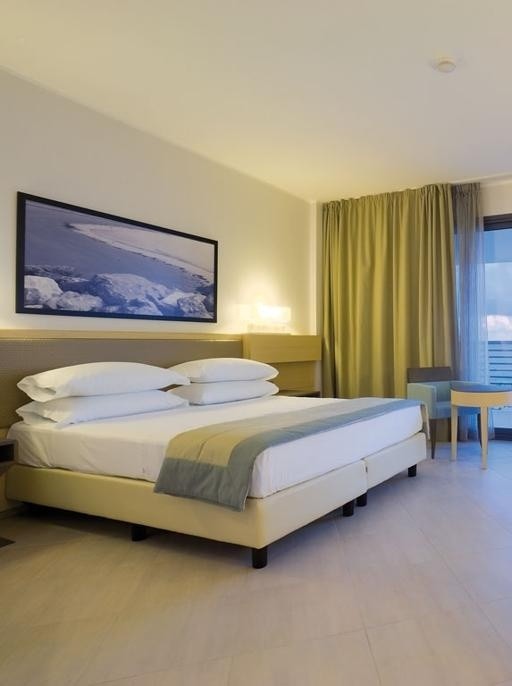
[450,383,512,470]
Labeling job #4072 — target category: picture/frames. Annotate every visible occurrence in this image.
[16,190,219,324]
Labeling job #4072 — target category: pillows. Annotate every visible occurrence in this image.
[17,357,281,427]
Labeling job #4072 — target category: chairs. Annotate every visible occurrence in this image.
[405,365,483,460]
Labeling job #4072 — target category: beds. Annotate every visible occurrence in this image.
[10,393,430,568]
